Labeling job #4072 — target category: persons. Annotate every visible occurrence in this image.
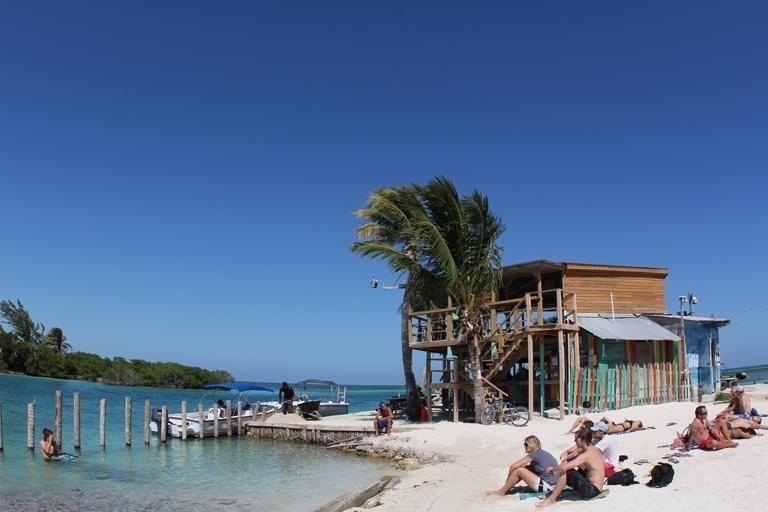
[418,386,425,397]
[37,427,57,460]
[486,416,643,508]
[242,404,250,410]
[432,388,442,402]
[217,399,225,408]
[373,401,393,436]
[686,385,768,452]
[279,382,295,415]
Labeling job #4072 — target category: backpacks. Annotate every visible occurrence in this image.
[650,461,675,487]
[608,468,640,486]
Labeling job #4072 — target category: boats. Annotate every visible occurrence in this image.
[150,379,349,438]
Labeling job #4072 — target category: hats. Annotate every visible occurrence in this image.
[590,421,609,433]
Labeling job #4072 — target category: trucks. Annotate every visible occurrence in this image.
[721,376,737,389]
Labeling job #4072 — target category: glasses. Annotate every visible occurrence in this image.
[699,411,707,415]
[524,443,529,446]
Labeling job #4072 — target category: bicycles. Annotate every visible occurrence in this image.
[481,393,530,427]
